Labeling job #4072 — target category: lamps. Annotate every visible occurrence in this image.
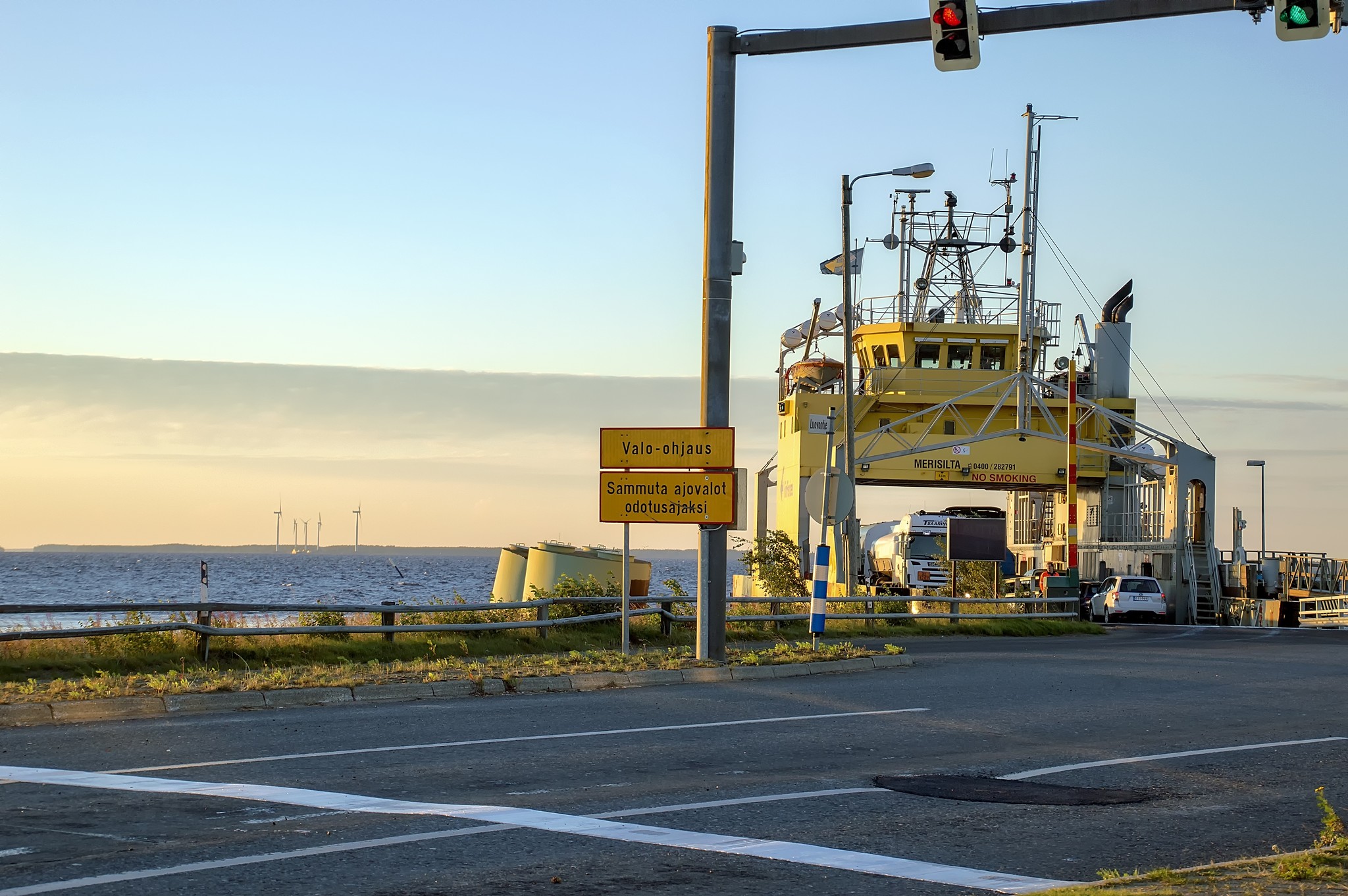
[860,463,870,472]
[968,293,982,310]
[1053,356,1070,378]
[1056,467,1067,478]
[913,276,930,295]
[961,467,970,477]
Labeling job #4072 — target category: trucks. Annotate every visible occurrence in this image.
[859,511,967,590]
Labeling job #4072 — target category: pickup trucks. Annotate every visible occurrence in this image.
[1020,569,1066,598]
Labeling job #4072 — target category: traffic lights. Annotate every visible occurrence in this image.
[1274,0,1332,40]
[928,0,981,71]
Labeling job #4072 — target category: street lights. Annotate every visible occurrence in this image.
[841,161,937,598]
[1246,460,1265,559]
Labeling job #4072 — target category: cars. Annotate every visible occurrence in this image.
[1089,575,1167,625]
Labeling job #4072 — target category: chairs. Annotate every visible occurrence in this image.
[1134,584,1144,592]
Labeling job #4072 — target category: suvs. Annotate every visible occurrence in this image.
[1079,577,1105,613]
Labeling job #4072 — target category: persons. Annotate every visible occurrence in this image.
[1039,562,1059,595]
